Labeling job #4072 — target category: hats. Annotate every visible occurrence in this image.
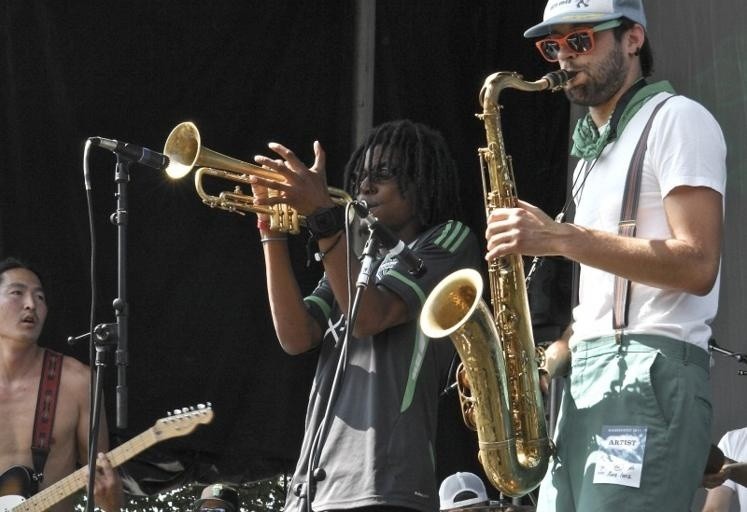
[522,0,647,39]
[437,472,488,510]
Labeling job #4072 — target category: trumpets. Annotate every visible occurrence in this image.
[162,122,369,235]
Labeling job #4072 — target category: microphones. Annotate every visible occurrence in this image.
[353,201,427,278]
[91,137,170,170]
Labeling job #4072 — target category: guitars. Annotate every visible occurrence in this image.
[0,401,215,512]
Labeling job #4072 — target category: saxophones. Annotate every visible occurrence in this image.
[418,69,577,498]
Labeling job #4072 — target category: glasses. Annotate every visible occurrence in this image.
[533,19,621,63]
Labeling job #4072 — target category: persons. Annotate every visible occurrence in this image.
[0,256,124,512]
[699,427,747,512]
[439,472,535,512]
[483,1,728,512]
[251,119,481,512]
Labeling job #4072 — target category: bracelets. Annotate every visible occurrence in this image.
[256,219,270,231]
[260,232,289,243]
[313,231,344,263]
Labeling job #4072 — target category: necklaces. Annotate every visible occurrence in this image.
[555,141,605,223]
